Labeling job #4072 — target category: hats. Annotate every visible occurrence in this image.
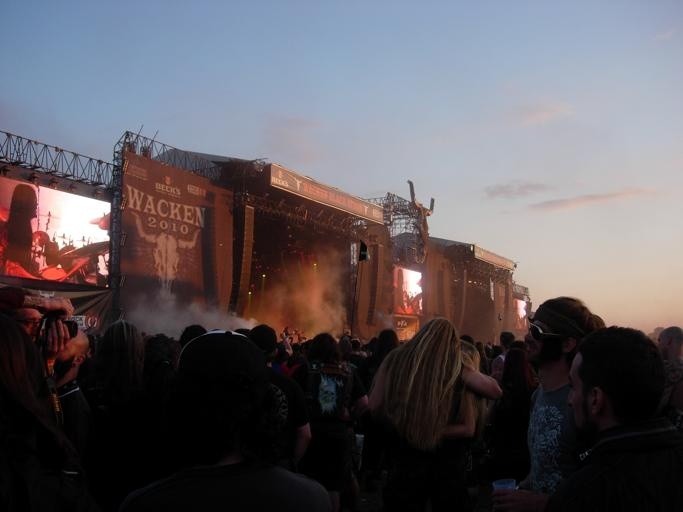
[177,330,267,382]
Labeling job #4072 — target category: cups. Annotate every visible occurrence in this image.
[492,479,516,493]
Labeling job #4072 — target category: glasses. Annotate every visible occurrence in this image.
[526,317,561,342]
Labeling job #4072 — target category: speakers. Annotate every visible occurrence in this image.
[456,269,467,328]
[504,286,512,330]
[365,244,385,324]
[228,205,255,317]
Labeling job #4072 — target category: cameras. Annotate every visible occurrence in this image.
[45,309,78,337]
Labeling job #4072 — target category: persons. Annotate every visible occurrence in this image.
[0,181,72,281]
[1,283,683,512]
[393,267,422,317]
